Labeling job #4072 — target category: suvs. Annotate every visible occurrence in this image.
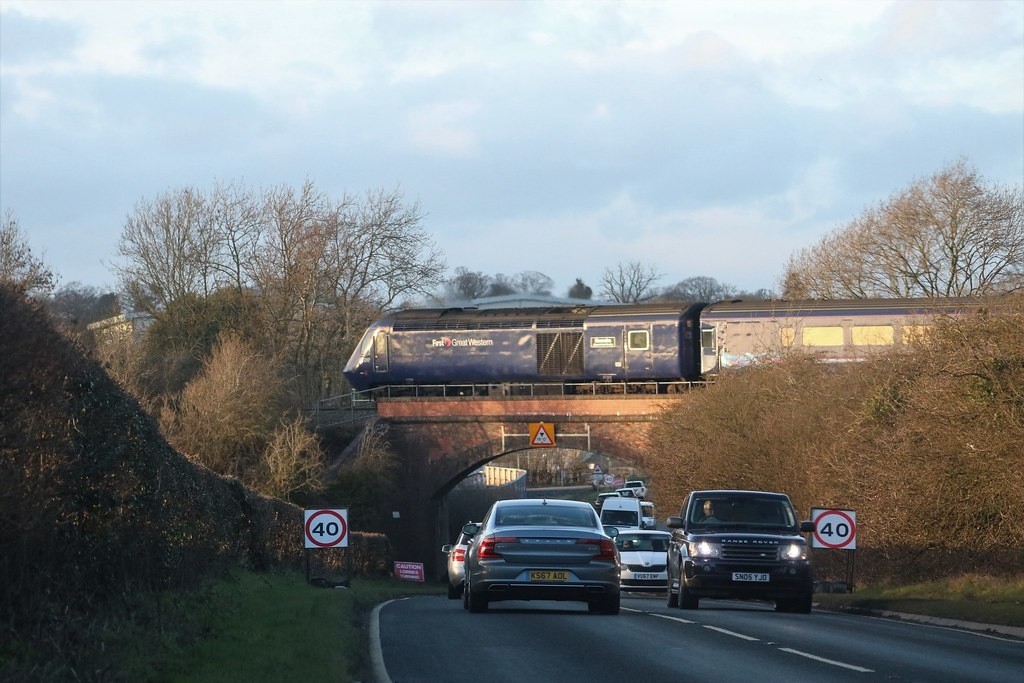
[667,490,816,613]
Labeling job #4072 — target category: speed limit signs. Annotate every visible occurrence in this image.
[809,506,858,548]
[303,508,348,547]
[603,474,615,484]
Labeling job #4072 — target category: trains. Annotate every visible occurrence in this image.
[342,299,1005,396]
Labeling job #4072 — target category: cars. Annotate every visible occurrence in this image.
[463,497,623,615]
[611,528,673,592]
[442,522,482,600]
[594,480,656,528]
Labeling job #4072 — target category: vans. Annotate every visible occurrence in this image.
[600,495,643,529]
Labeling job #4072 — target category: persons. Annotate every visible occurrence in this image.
[703,500,717,517]
[623,541,631,547]
